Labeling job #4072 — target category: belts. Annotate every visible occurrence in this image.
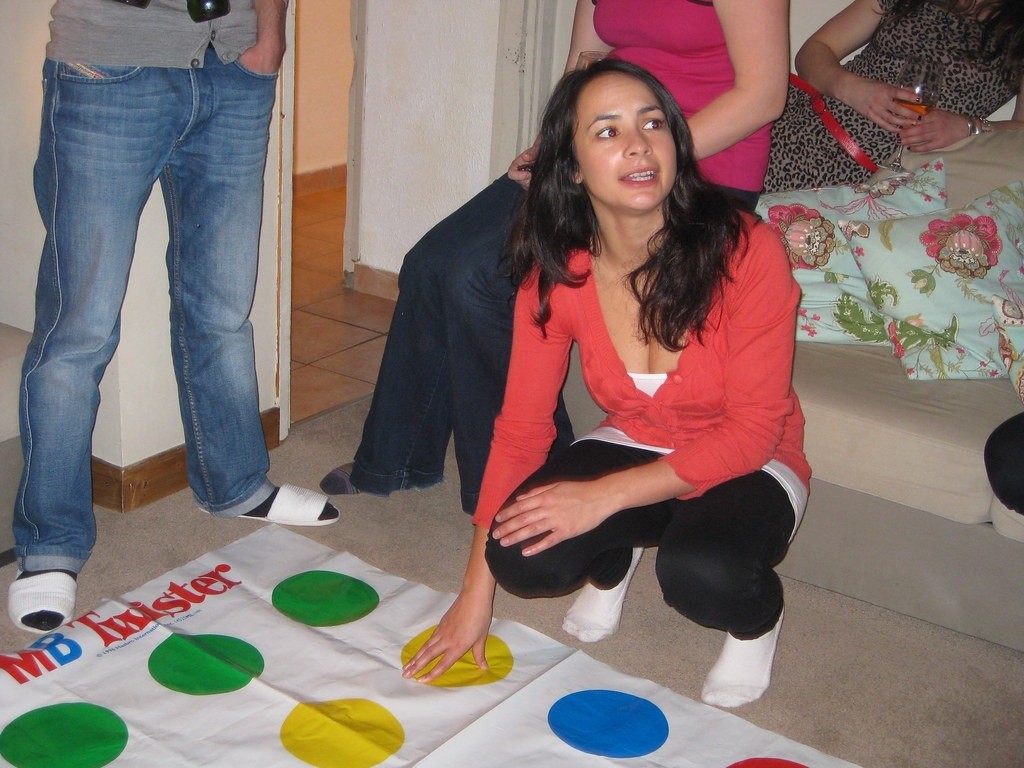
[787,71,878,174]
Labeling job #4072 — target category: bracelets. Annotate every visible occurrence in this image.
[962,113,993,136]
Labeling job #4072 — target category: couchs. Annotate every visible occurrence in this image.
[560,128,1023,650]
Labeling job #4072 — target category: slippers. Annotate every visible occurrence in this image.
[198,483,341,528]
[8,568,78,634]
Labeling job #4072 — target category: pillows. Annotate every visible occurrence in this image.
[836,179,1024,379]
[755,157,949,346]
[992,295,1024,412]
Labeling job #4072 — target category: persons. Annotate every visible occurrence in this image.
[762,0,1024,192]
[399,53,813,707]
[10,0,340,632]
[321,0,790,521]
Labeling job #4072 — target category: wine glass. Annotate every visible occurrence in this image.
[877,53,944,178]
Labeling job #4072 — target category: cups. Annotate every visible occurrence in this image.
[575,51,619,72]
